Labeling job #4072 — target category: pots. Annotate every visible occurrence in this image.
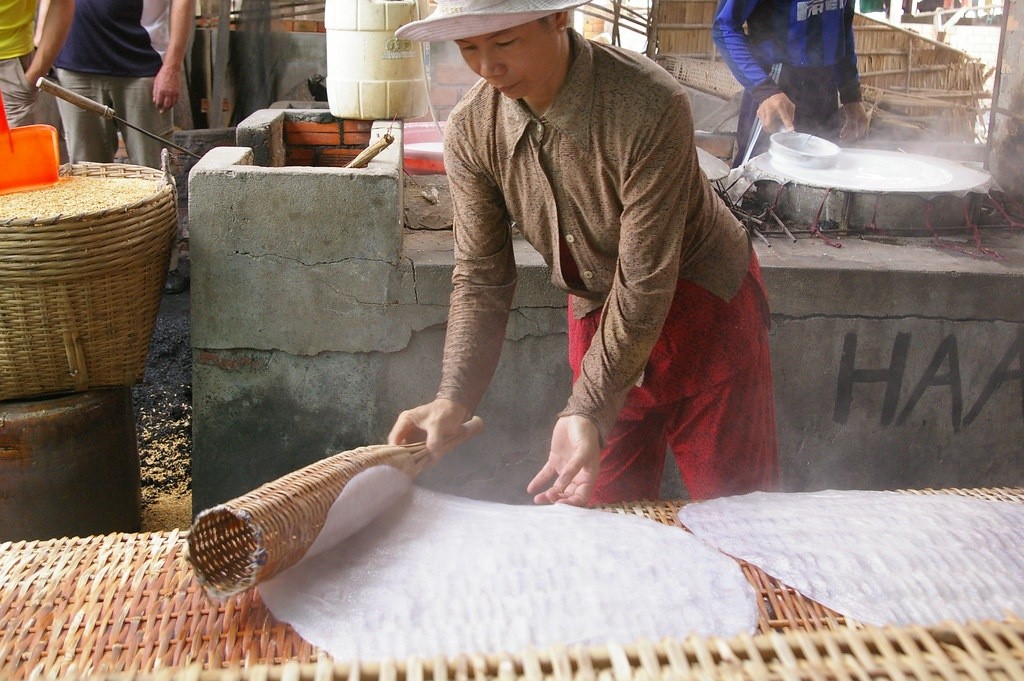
[746,148,991,233]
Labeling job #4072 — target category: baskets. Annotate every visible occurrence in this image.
[0,162,177,399]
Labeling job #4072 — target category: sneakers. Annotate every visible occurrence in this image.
[165,270,188,293]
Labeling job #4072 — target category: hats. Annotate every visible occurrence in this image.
[395,0,596,42]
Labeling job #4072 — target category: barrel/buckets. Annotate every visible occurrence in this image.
[324,0,430,120]
[404,127,448,174]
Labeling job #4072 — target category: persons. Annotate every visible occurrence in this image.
[1,1,197,171]
[711,0,869,169]
[388,0,780,508]
